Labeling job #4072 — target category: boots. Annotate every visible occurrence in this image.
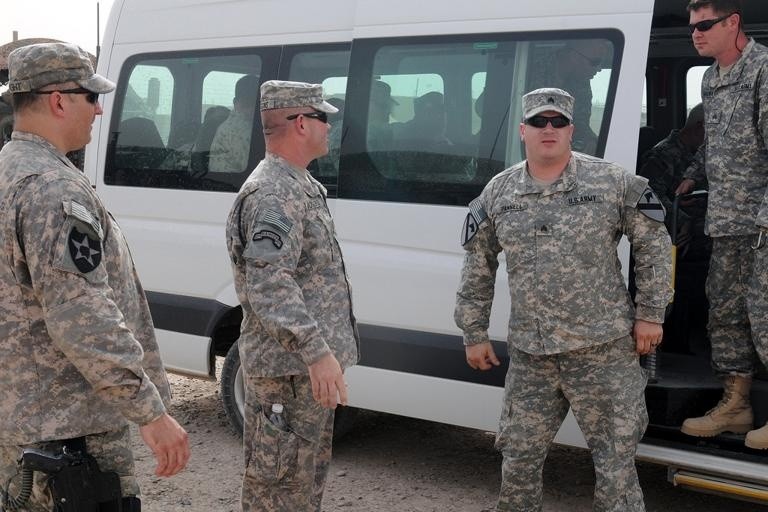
[743,419,768,451]
[680,374,755,438]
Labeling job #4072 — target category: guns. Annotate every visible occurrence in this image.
[19,446,122,512]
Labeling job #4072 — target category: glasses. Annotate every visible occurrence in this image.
[522,115,571,129]
[687,13,735,35]
[36,86,101,105]
[286,111,328,124]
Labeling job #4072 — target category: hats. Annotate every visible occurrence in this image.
[520,87,575,124]
[369,80,401,106]
[258,79,340,115]
[413,91,448,113]
[5,41,117,95]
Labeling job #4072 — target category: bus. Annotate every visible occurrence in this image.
[81,0,767,504]
